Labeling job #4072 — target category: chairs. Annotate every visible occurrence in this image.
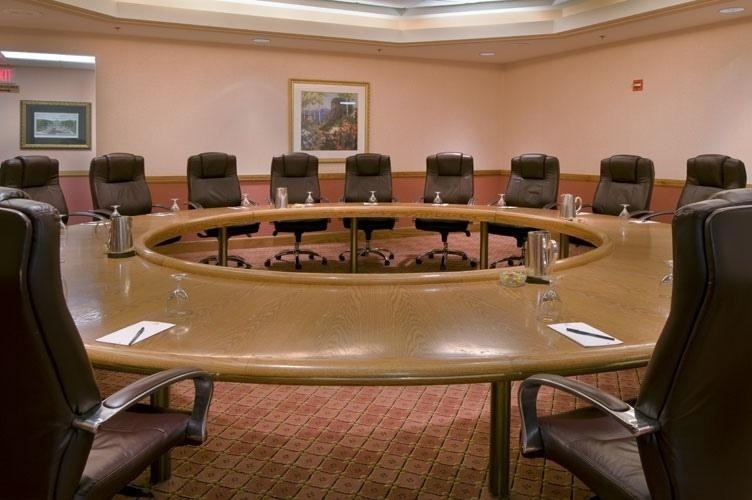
[263,153,332,270]
[0,198,211,498]
[89,153,181,248]
[486,154,561,273]
[1,154,106,227]
[184,153,262,266]
[339,154,399,266]
[515,188,747,498]
[635,152,748,224]
[570,156,654,220]
[412,151,476,271]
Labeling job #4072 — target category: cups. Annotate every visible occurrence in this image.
[499,269,526,286]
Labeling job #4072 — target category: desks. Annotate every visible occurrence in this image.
[42,204,673,500]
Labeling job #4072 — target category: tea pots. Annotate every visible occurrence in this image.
[275,188,288,208]
[108,216,136,258]
[559,193,582,219]
[525,230,559,284]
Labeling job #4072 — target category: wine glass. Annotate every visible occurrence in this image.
[305,192,314,207]
[618,203,630,222]
[496,193,506,209]
[170,197,180,214]
[434,192,442,205]
[369,191,376,203]
[58,213,67,243]
[166,274,191,335]
[538,275,562,324]
[110,205,121,220]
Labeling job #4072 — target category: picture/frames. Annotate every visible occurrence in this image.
[288,78,371,163]
[20,100,92,150]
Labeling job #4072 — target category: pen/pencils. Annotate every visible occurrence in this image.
[567,327,614,340]
[128,327,144,346]
